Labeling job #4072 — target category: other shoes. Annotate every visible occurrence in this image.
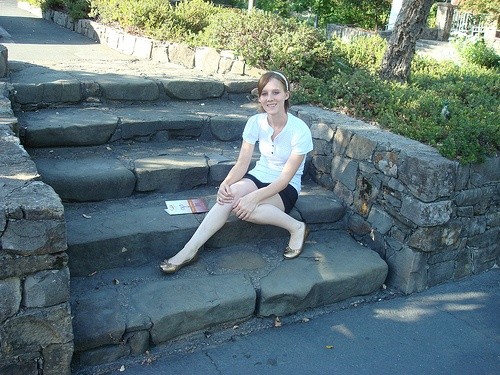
[282,221,309,260]
[158,250,198,275]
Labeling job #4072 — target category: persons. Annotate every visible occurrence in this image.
[158,70,312,276]
